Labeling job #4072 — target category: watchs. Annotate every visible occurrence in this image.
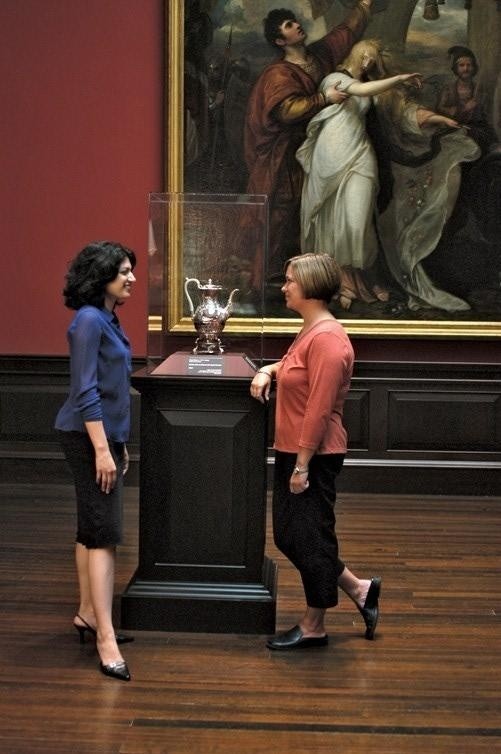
[292,465,309,476]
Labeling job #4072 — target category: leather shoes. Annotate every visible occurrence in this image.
[359,576,382,639]
[268,625,329,650]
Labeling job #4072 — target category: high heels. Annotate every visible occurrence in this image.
[99,660,131,681]
[72,614,134,645]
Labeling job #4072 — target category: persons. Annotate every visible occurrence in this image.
[249,252,382,651]
[52,239,138,681]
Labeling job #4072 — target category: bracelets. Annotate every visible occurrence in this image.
[256,370,271,379]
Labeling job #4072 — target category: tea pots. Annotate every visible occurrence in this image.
[183,277,240,355]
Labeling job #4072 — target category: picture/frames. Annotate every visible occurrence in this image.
[159,0,501,343]
